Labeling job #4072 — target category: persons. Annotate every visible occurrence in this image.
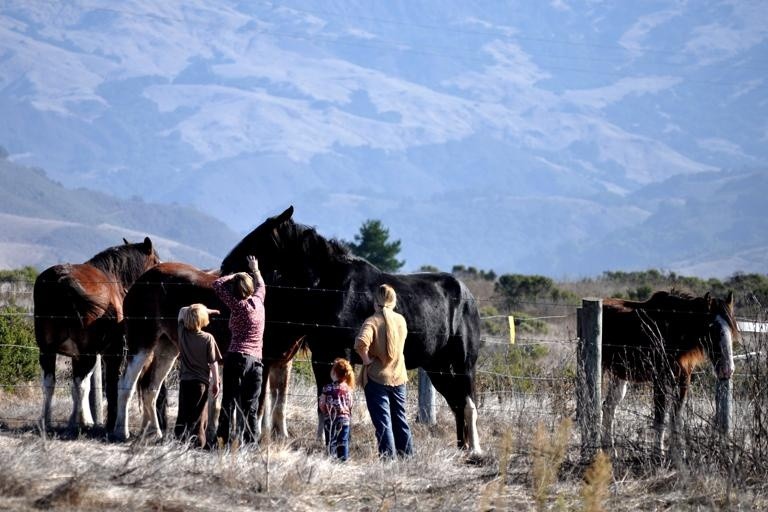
[318,358,356,464]
[169,302,224,451]
[213,251,266,448]
[353,283,416,460]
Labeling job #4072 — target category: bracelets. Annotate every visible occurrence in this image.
[250,270,260,275]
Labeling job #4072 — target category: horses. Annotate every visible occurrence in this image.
[602,286,740,465]
[111,260,307,443]
[33,235,164,434]
[219,205,490,466]
